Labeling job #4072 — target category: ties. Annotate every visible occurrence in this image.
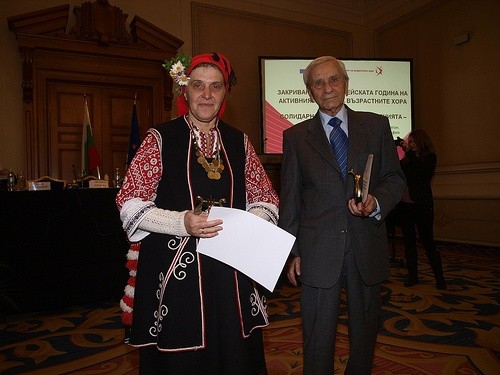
[327,117,349,184]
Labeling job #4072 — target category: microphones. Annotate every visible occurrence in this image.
[72,165,78,183]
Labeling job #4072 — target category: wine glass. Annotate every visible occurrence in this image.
[77,169,87,189]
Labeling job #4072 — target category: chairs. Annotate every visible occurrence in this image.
[72,175,99,188]
[0,167,25,189]
[26,175,67,191]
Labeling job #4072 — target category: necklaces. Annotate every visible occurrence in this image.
[192,130,225,180]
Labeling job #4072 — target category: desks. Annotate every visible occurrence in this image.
[0,186,131,315]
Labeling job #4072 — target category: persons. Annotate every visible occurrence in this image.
[277,57,408,375]
[399,129,446,290]
[112,53,280,375]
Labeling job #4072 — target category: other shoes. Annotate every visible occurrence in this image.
[435,278,448,289]
[403,275,418,287]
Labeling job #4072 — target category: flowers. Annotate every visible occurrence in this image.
[161,52,193,96]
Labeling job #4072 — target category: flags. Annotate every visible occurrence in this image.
[123,99,142,170]
[82,96,101,177]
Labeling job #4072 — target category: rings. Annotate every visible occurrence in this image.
[202,228,204,234]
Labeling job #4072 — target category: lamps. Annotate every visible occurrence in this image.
[454,32,470,46]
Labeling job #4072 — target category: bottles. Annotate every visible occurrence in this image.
[113,167,121,188]
[7,168,26,192]
